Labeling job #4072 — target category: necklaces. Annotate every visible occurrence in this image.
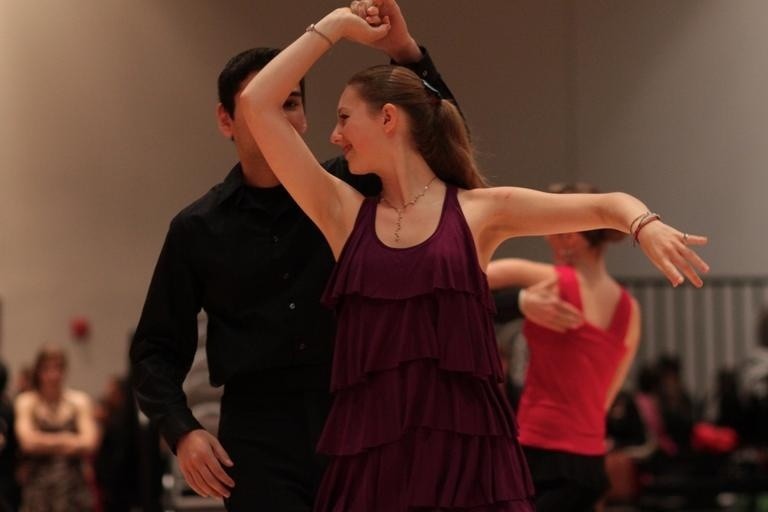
[380,176,437,242]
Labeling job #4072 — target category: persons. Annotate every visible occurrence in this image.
[240,0,710,511]
[483,183,641,510]
[489,273,584,334]
[129,1,471,511]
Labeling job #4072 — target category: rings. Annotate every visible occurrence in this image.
[682,233,689,245]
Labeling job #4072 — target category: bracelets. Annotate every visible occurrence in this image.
[306,23,335,49]
[629,211,662,246]
